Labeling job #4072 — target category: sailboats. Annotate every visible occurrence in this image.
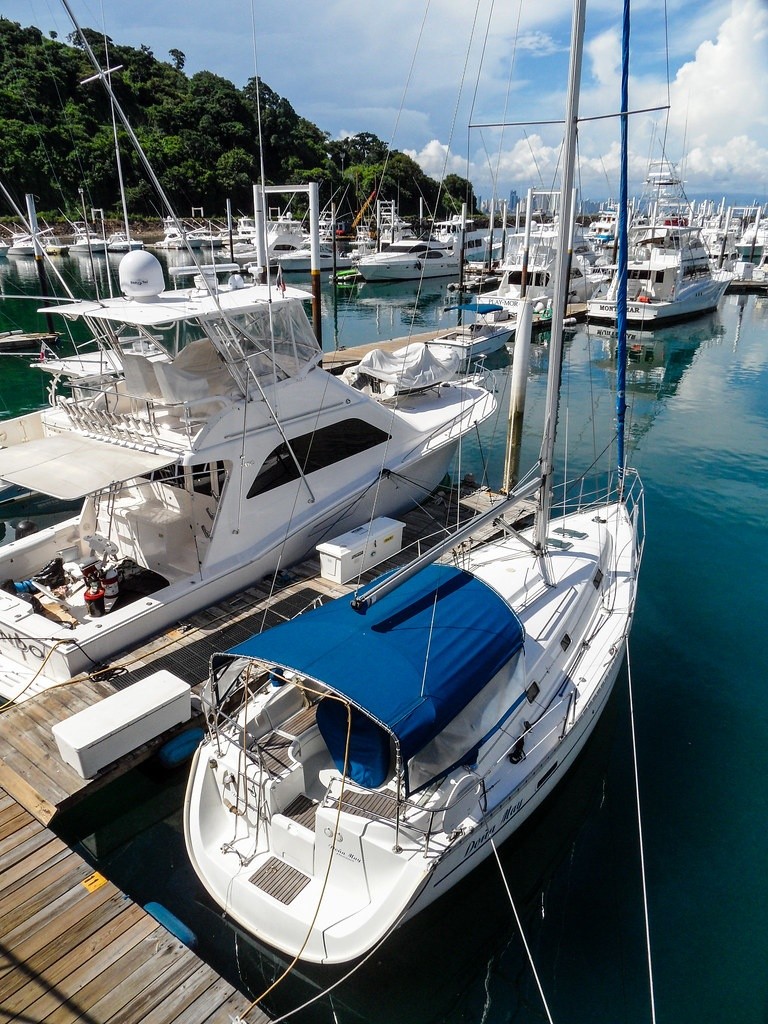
[1,1,768,967]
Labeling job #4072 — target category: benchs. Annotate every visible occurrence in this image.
[487,309,508,323]
[249,704,328,809]
[331,774,427,823]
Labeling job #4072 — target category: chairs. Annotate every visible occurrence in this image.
[153,361,210,434]
[122,354,163,422]
